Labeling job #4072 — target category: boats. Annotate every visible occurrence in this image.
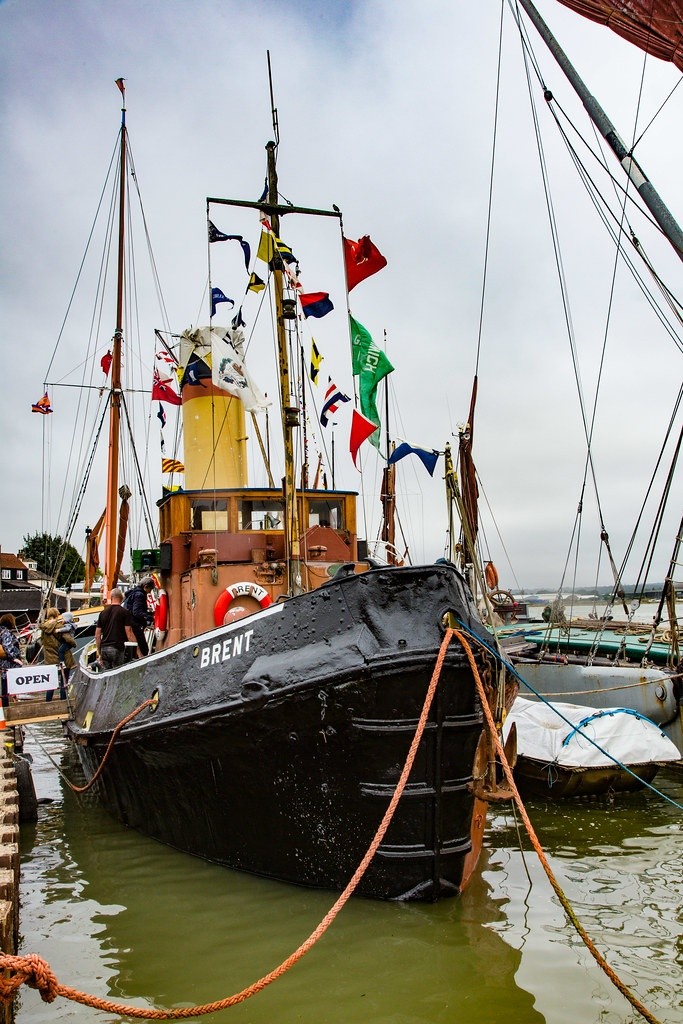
[494,696,681,796]
[501,154,682,727]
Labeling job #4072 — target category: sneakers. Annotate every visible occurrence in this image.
[57,662,67,671]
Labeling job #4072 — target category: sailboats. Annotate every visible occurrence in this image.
[16,44,512,910]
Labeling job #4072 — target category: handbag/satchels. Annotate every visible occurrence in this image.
[0,645,8,659]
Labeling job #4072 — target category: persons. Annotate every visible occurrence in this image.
[40,607,77,702]
[94,588,144,670]
[0,614,23,708]
[55,611,77,660]
[123,576,154,657]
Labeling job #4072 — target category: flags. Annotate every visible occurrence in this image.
[149,177,440,497]
[31,392,53,414]
[100,351,112,376]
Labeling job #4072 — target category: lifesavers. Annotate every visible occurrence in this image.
[154,589,168,641]
[485,562,498,590]
[213,580,277,629]
